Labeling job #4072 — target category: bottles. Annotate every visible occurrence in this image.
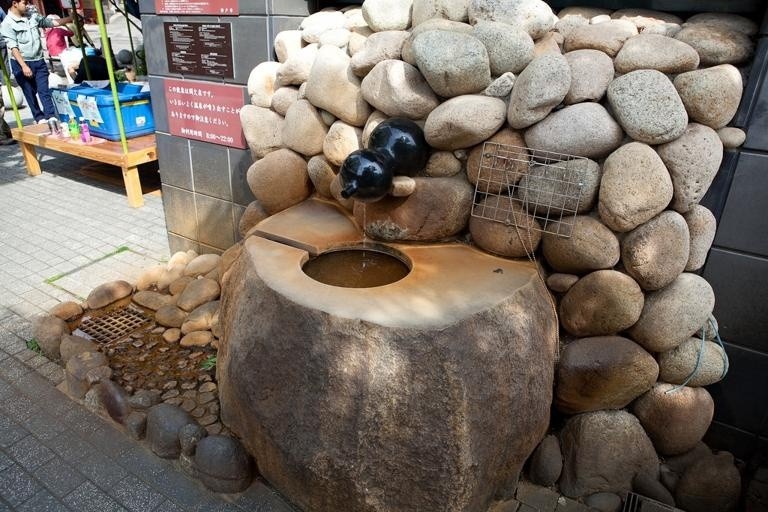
[59,120,70,137]
[338,118,428,203]
[68,118,80,140]
[79,117,92,144]
[47,118,61,135]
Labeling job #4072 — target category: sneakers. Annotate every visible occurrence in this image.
[37,118,47,124]
[48,117,57,121]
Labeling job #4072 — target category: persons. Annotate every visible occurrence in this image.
[0,1,137,146]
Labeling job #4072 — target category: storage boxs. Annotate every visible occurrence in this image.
[49,82,155,143]
